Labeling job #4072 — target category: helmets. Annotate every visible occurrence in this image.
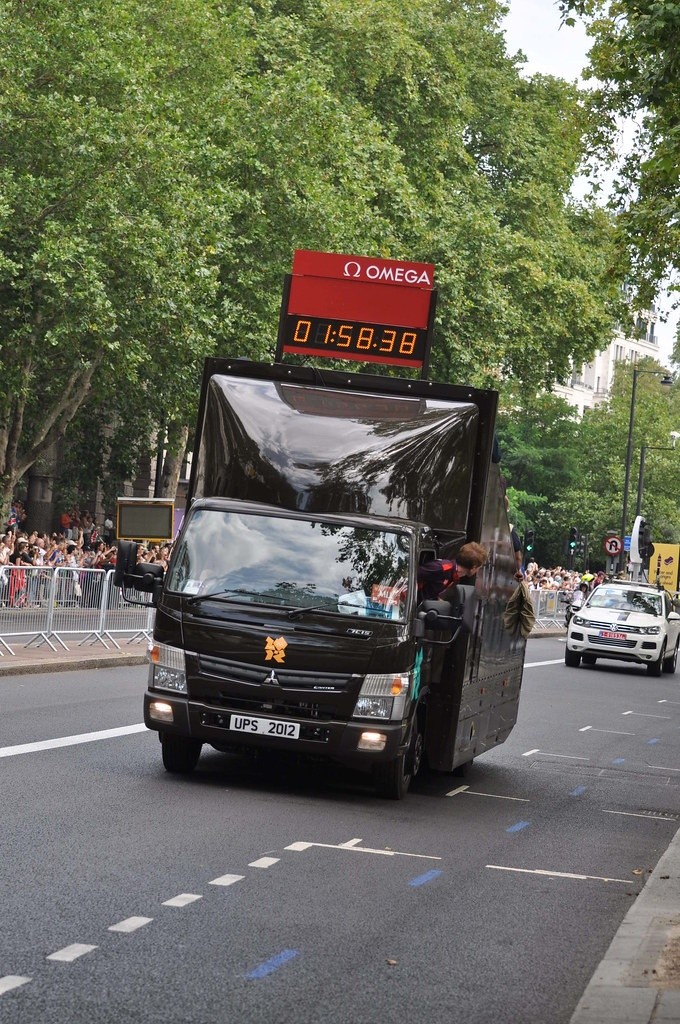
[579,580,589,592]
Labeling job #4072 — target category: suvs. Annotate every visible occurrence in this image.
[564,579,680,676]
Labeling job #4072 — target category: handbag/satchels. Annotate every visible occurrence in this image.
[74,581,82,596]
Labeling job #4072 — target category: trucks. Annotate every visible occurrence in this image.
[113,250,536,800]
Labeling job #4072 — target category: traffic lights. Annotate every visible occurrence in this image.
[568,527,577,556]
[525,530,534,554]
[638,520,652,558]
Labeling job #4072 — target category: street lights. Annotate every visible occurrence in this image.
[617,370,675,575]
[635,430,680,517]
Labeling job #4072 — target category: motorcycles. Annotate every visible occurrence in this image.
[561,590,586,628]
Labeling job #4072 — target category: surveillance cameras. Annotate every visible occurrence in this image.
[670,431,679,439]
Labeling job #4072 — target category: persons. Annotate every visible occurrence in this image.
[504,495,536,637]
[525,557,627,626]
[0,499,177,610]
[388,540,489,609]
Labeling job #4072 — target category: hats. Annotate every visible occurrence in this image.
[17,537,28,544]
[555,575,561,581]
[68,540,77,546]
[84,546,92,551]
